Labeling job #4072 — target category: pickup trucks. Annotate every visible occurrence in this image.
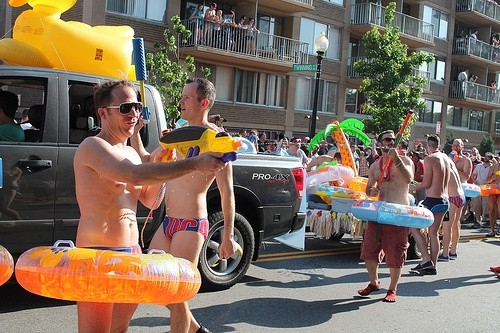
[0,64,307,291]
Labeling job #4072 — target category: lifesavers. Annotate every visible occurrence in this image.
[351,199,435,230]
[478,183,500,196]
[14,244,202,305]
[315,185,365,200]
[306,164,354,195]
[461,181,481,199]
[0,243,15,289]
[348,175,379,196]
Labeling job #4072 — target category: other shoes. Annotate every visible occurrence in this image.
[496,228,500,235]
[474,222,480,227]
[466,214,475,222]
[411,259,434,270]
[449,251,457,259]
[460,219,469,224]
[196,325,211,333]
[486,232,495,237]
[414,268,437,275]
[383,289,397,302]
[482,221,496,229]
[438,252,449,261]
[358,282,380,296]
[490,266,500,272]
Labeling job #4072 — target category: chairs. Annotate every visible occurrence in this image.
[24,105,44,142]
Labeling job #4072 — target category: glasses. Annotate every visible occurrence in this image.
[381,138,396,142]
[102,102,144,115]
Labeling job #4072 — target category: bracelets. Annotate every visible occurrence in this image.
[396,161,402,167]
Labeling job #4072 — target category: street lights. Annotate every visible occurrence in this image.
[310,31,329,140]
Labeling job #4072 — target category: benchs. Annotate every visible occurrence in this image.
[70,116,101,144]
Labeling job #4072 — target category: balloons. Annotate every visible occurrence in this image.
[8,0,135,80]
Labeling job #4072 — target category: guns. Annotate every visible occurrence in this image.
[158,125,243,164]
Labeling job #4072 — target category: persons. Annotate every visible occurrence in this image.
[448,139,472,183]
[350,141,444,207]
[0,91,39,142]
[458,69,500,104]
[189,2,260,54]
[437,154,466,261]
[412,144,500,238]
[357,130,415,302]
[130,78,236,333]
[469,0,500,21]
[410,134,451,276]
[454,29,500,63]
[74,81,228,333]
[0,167,23,220]
[227,128,350,170]
